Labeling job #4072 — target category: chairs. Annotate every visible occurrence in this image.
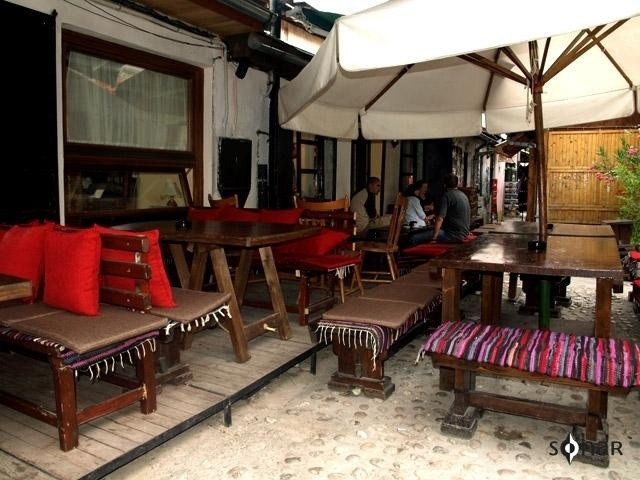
[1,228,161,451]
[65,224,220,389]
[208,195,239,209]
[349,193,406,289]
[206,209,364,319]
[292,194,364,304]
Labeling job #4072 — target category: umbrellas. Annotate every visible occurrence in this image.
[273,0,639,331]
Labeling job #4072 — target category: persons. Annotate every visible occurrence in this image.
[417,180,437,218]
[348,176,384,274]
[410,174,470,247]
[403,182,428,231]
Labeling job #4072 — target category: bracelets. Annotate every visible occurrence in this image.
[431,238,437,241]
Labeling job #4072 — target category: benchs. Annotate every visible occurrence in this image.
[405,226,480,261]
[413,313,639,466]
[320,257,462,399]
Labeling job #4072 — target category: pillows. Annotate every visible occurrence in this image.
[43,227,103,316]
[276,226,350,257]
[188,209,264,226]
[256,208,304,224]
[1,221,49,306]
[92,222,179,309]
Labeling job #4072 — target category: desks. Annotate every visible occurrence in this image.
[487,219,620,326]
[438,237,626,395]
[107,217,325,363]
